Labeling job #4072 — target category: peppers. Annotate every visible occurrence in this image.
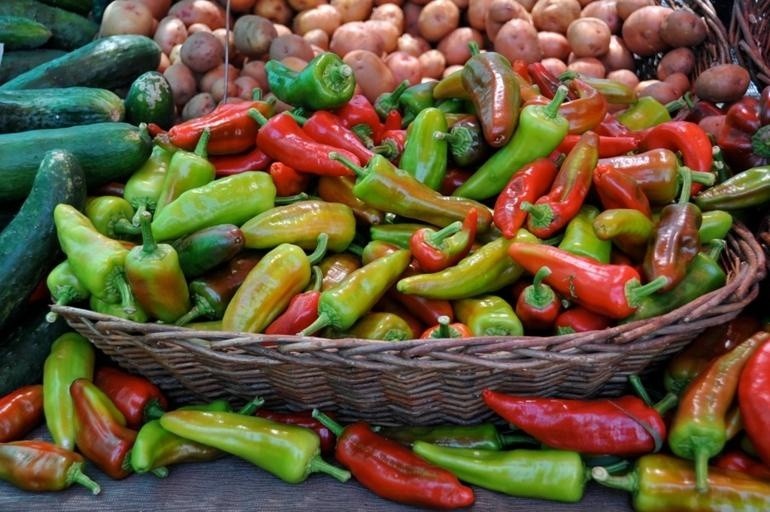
[2,41,770,511]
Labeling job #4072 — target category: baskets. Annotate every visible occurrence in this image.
[728,1,770,94]
[623,0,733,123]
[50,219,766,434]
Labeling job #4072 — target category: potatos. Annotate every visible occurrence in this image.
[96,1,753,119]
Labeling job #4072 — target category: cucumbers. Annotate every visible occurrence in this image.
[0,1,175,398]
